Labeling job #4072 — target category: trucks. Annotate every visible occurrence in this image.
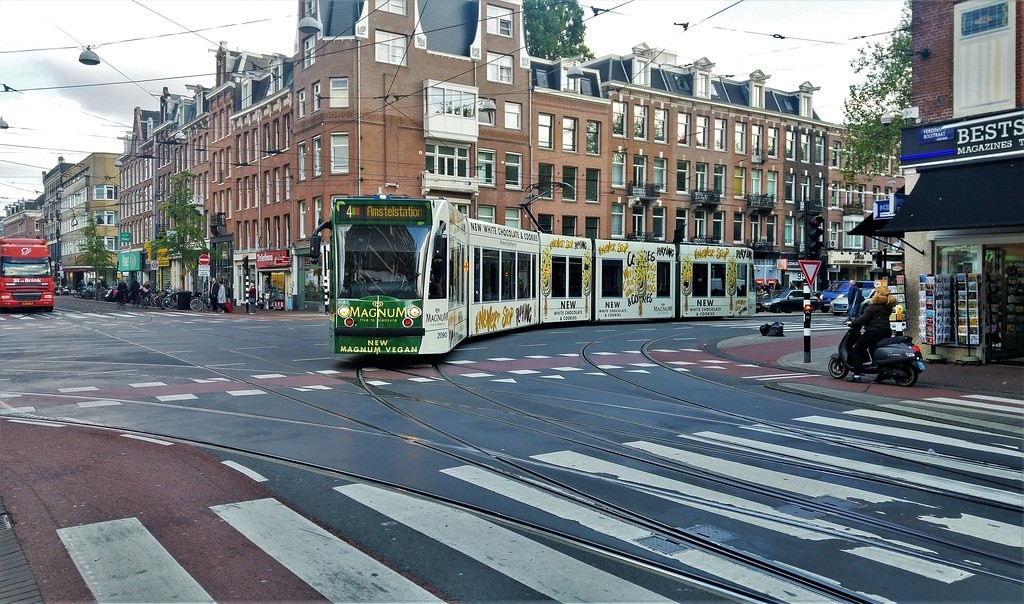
[0,238,56,311]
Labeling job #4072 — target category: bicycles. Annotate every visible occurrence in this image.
[188,289,225,313]
[72,286,179,310]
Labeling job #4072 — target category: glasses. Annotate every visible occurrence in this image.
[874,291,878,293]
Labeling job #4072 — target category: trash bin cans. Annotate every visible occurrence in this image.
[177,291,191,310]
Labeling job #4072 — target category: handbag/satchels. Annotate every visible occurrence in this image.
[249,298,256,303]
[859,296,865,304]
[143,287,149,292]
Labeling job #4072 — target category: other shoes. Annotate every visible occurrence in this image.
[250,313,256,314]
[847,375,861,383]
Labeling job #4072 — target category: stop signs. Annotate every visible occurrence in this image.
[198,255,210,265]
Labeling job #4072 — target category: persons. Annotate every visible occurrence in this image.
[845,280,860,321]
[430,276,442,297]
[482,284,493,300]
[117,279,152,309]
[217,281,226,313]
[517,289,523,298]
[96,276,117,290]
[247,283,257,314]
[986,261,997,326]
[78,279,94,291]
[210,277,219,312]
[756,284,770,298]
[845,286,897,384]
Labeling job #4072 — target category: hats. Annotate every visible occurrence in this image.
[850,280,854,285]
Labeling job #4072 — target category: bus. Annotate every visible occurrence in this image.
[310,181,756,362]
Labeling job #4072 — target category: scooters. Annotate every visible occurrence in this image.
[829,318,926,387]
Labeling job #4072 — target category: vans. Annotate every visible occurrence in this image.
[822,280,876,313]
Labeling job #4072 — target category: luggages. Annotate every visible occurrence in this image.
[225,301,234,313]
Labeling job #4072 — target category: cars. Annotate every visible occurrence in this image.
[829,287,873,316]
[755,289,820,313]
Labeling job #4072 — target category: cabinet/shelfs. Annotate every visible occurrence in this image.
[982,263,1024,363]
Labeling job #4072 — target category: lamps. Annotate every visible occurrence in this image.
[564,61,587,82]
[113,159,124,168]
[57,185,66,193]
[76,46,103,68]
[0,115,10,130]
[174,129,188,142]
[295,14,322,37]
[482,99,498,114]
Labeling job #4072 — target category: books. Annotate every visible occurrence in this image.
[918,273,982,346]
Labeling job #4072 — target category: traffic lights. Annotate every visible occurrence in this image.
[242,256,249,269]
[804,211,830,254]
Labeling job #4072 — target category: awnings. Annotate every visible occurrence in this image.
[826,249,872,267]
[847,183,905,238]
[876,153,1024,232]
